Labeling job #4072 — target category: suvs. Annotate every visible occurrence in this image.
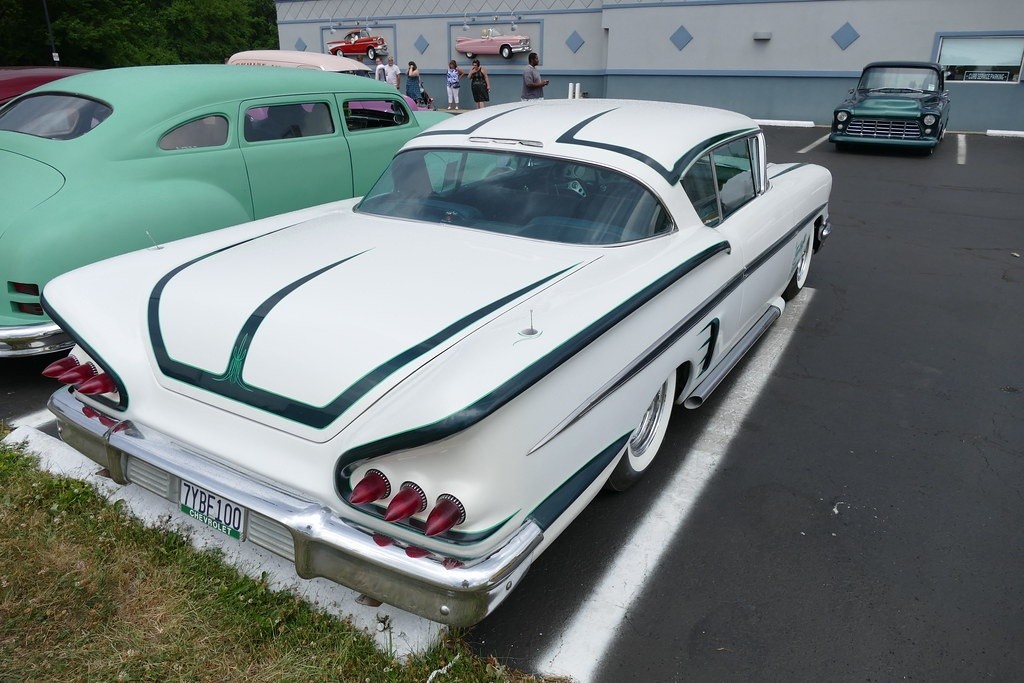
[829,59,954,159]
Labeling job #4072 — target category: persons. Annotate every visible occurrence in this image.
[384,56,400,90]
[405,61,422,107]
[374,57,385,81]
[466,60,491,109]
[447,60,464,110]
[521,53,549,101]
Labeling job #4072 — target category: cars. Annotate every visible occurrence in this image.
[227,50,376,83]
[325,28,389,61]
[0,67,428,147]
[454,28,533,59]
[39,98,834,633]
[0,61,522,377]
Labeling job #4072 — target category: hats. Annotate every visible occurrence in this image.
[450,60,456,65]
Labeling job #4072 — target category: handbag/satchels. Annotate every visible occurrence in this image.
[453,83,460,88]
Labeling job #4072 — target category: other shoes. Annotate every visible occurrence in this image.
[448,107,452,109]
[455,107,458,110]
